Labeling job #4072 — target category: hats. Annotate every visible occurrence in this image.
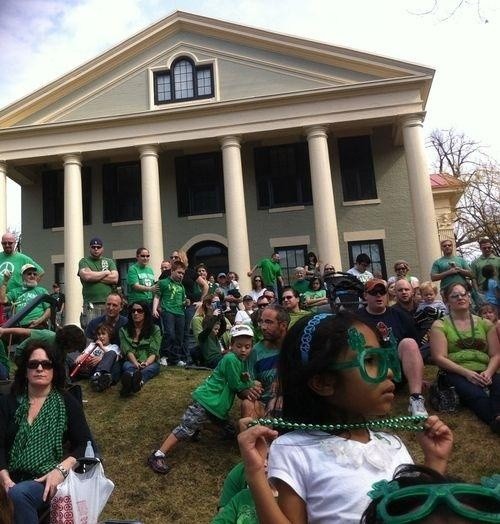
[53,284,60,288]
[243,295,253,301]
[90,237,103,246]
[363,277,388,292]
[218,273,226,278]
[20,263,37,274]
[230,324,254,338]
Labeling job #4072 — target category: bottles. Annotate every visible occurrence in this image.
[84,440,95,473]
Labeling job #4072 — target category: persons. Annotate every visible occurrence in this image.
[0,233,499,524]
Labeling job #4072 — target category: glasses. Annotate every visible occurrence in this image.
[339,345,403,384]
[375,482,500,523]
[450,290,467,298]
[91,246,101,249]
[443,244,451,247]
[141,255,150,257]
[132,309,143,313]
[367,288,386,296]
[258,303,268,308]
[281,296,292,300]
[170,256,178,259]
[255,280,261,282]
[1,241,13,245]
[26,359,53,370]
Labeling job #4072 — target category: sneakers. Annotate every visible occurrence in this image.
[160,356,168,366]
[132,370,142,393]
[408,394,429,418]
[176,361,186,368]
[119,370,132,398]
[147,450,171,474]
[91,373,113,392]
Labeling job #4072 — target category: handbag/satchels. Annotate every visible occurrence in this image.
[430,386,459,413]
